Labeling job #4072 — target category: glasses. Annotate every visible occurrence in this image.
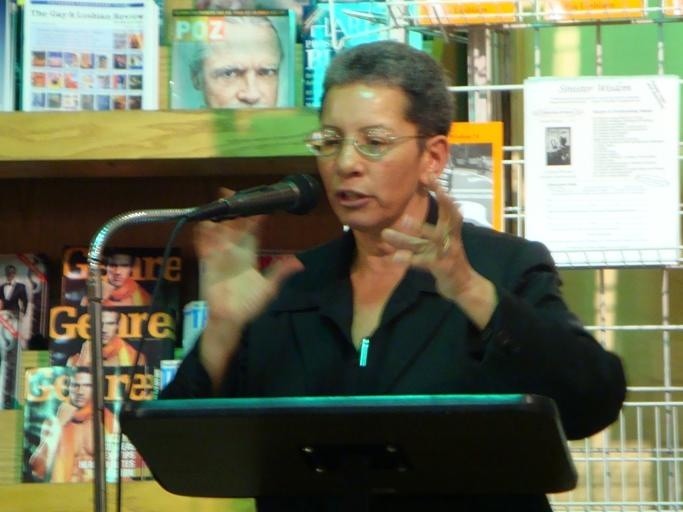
[303,129,428,157]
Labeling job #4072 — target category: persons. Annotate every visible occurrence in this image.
[188,14,283,108]
[28,247,153,483]
[118,40,628,511]
[0,265,29,315]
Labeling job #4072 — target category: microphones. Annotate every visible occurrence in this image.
[185,173,323,223]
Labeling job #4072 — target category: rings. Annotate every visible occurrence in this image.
[441,237,450,250]
